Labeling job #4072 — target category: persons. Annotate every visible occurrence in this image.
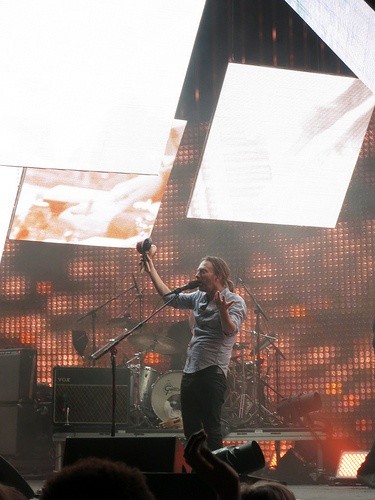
[138,251,246,451]
[0,429,295,500]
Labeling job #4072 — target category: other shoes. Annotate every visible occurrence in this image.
[356,474,375,488]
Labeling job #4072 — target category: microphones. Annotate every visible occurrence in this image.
[170,401,177,407]
[130,273,140,293]
[162,281,198,297]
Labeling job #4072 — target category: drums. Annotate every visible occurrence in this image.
[146,369,183,428]
[121,364,162,410]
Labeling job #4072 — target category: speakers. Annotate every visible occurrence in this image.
[0,348,38,458]
[53,366,133,428]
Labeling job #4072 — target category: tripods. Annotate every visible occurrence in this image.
[220,348,282,425]
[124,351,154,429]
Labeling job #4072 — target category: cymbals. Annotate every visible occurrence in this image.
[127,333,185,355]
[106,317,142,329]
